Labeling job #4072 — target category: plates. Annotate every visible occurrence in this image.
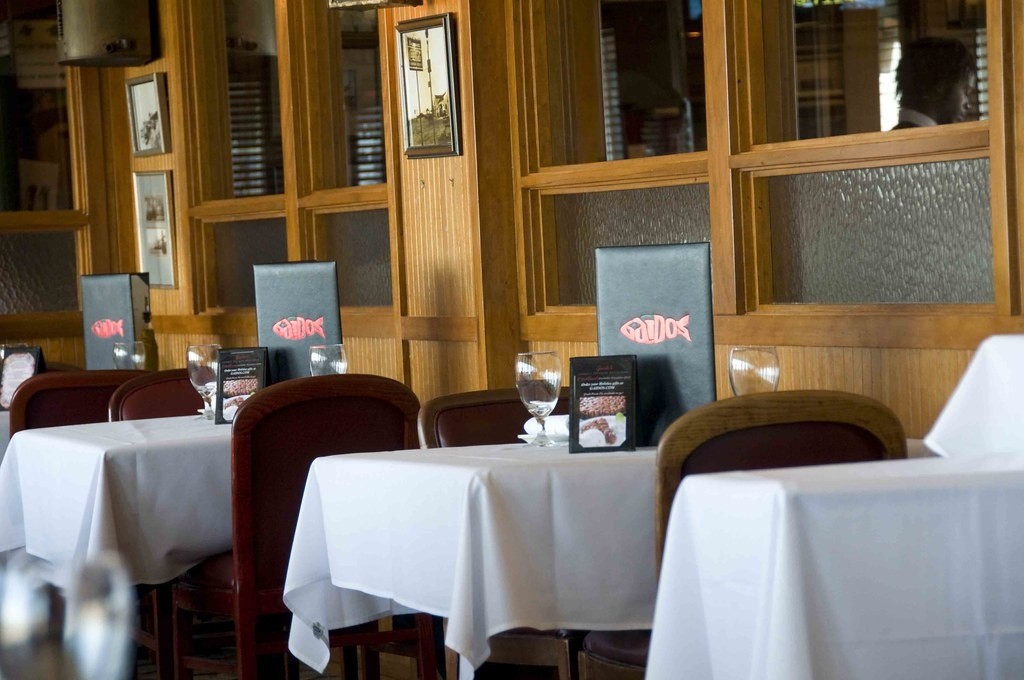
[516,432,535,444]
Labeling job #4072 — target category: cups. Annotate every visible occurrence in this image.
[112,341,146,371]
[310,343,347,378]
[729,346,779,396]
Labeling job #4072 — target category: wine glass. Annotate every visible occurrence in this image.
[185,344,220,421]
[516,352,560,448]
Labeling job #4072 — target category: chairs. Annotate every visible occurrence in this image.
[412,374,910,679]
[168,372,447,679]
[6,367,220,680]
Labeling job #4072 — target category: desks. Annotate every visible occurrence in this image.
[679,452,1024,680]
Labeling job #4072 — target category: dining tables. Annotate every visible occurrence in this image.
[318,433,663,680]
[13,413,231,680]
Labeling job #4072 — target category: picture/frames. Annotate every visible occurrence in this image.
[130,169,180,291]
[393,11,466,160]
[124,72,172,160]
